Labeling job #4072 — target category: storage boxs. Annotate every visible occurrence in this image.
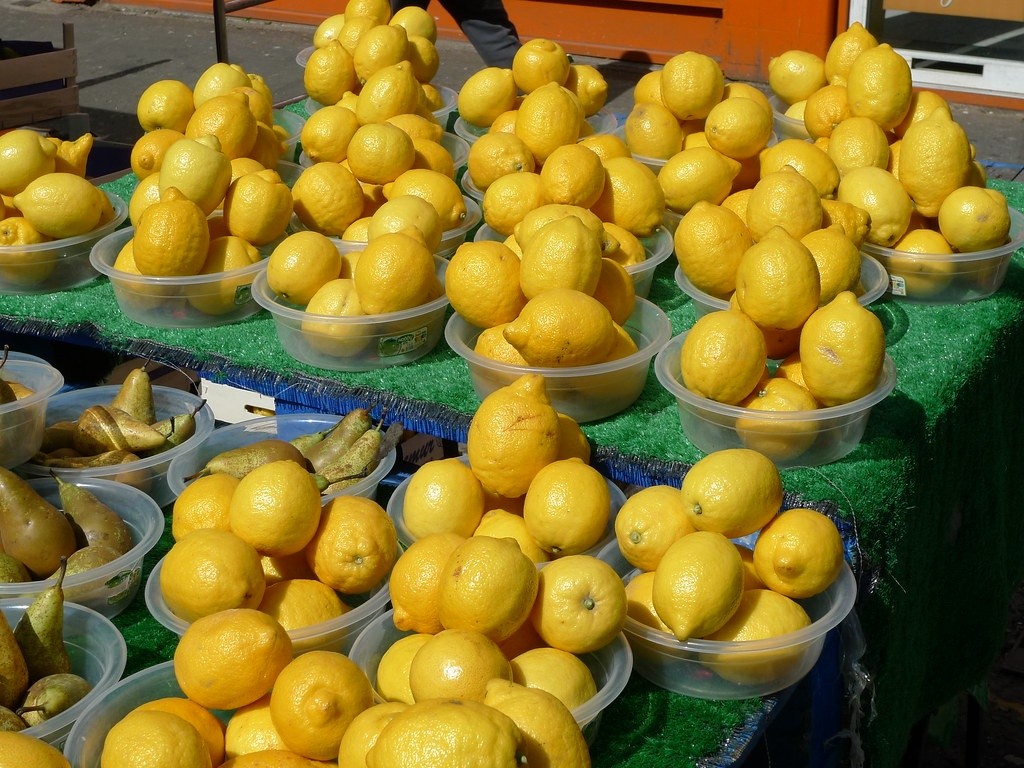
[0,23,81,131]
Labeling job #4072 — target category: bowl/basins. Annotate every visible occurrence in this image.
[0,45,1024,466]
[1,349,855,768]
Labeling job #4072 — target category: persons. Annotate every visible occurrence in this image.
[392,0,574,96]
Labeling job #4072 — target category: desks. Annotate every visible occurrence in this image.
[0,92,1024,768]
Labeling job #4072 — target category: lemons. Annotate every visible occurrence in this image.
[0,0,1014,462]
[0,730,72,768]
[96,375,847,768]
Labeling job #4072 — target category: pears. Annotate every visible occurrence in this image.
[0,345,386,731]
